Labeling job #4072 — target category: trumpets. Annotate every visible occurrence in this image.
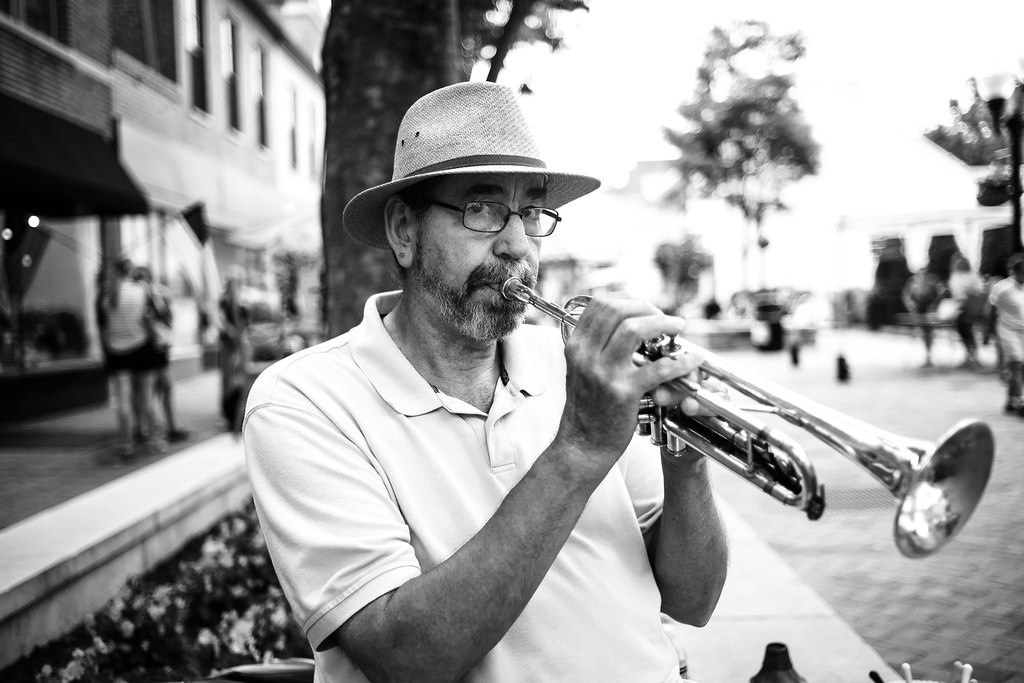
[500,276,993,559]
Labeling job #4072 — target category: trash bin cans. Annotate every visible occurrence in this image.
[755,305,786,351]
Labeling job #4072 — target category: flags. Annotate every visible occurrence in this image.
[183,205,211,246]
[6,220,48,305]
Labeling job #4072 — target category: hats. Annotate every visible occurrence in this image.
[344,82,601,250]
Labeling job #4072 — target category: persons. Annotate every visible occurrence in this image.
[655,241,721,321]
[217,277,248,417]
[96,260,190,460]
[868,236,1024,418]
[242,80,728,682]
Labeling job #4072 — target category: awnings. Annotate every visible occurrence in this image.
[0,94,153,221]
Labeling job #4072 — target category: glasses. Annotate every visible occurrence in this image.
[432,199,561,236]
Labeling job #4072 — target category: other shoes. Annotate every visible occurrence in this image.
[167,430,190,442]
